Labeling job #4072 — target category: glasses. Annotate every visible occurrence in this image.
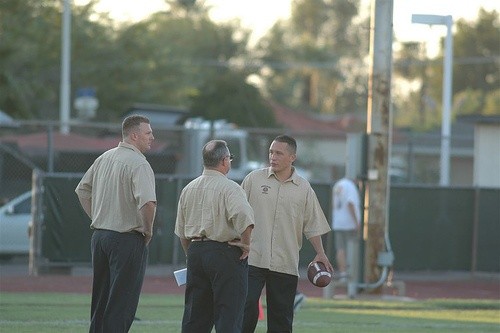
[227,155,234,163]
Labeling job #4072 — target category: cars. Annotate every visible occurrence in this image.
[0,189,33,257]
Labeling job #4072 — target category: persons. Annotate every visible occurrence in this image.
[331,176,362,284]
[174,139,255,333]
[240,135,334,333]
[74,114,158,333]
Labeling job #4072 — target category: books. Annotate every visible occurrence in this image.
[173,268,187,287]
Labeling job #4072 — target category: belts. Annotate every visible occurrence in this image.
[191,236,210,241]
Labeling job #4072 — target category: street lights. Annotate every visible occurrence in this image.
[411,13,454,188]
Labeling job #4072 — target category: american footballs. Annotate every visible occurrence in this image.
[307,260,332,287]
